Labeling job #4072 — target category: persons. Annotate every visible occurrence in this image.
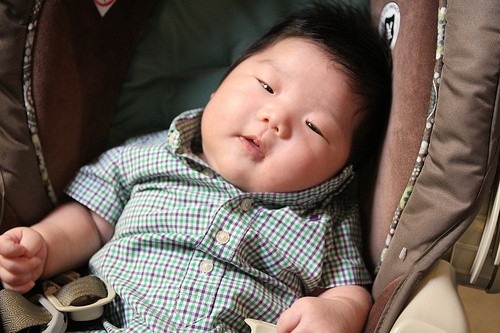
[2,0,375,333]
